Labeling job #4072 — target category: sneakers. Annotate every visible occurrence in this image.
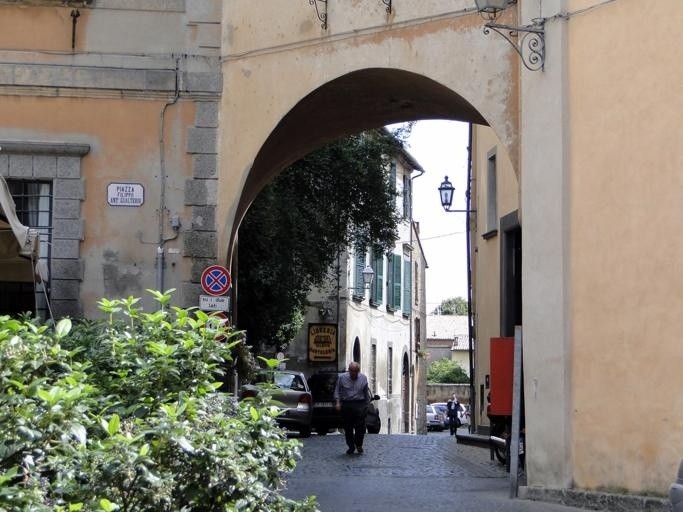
[346,446,363,455]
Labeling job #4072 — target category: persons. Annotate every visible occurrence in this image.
[446,392,460,436]
[460,397,471,434]
[334,362,372,454]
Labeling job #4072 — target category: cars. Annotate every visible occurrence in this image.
[426,401,472,429]
[241,371,381,434]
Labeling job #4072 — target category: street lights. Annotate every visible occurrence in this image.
[477,2,546,71]
[348,266,374,289]
